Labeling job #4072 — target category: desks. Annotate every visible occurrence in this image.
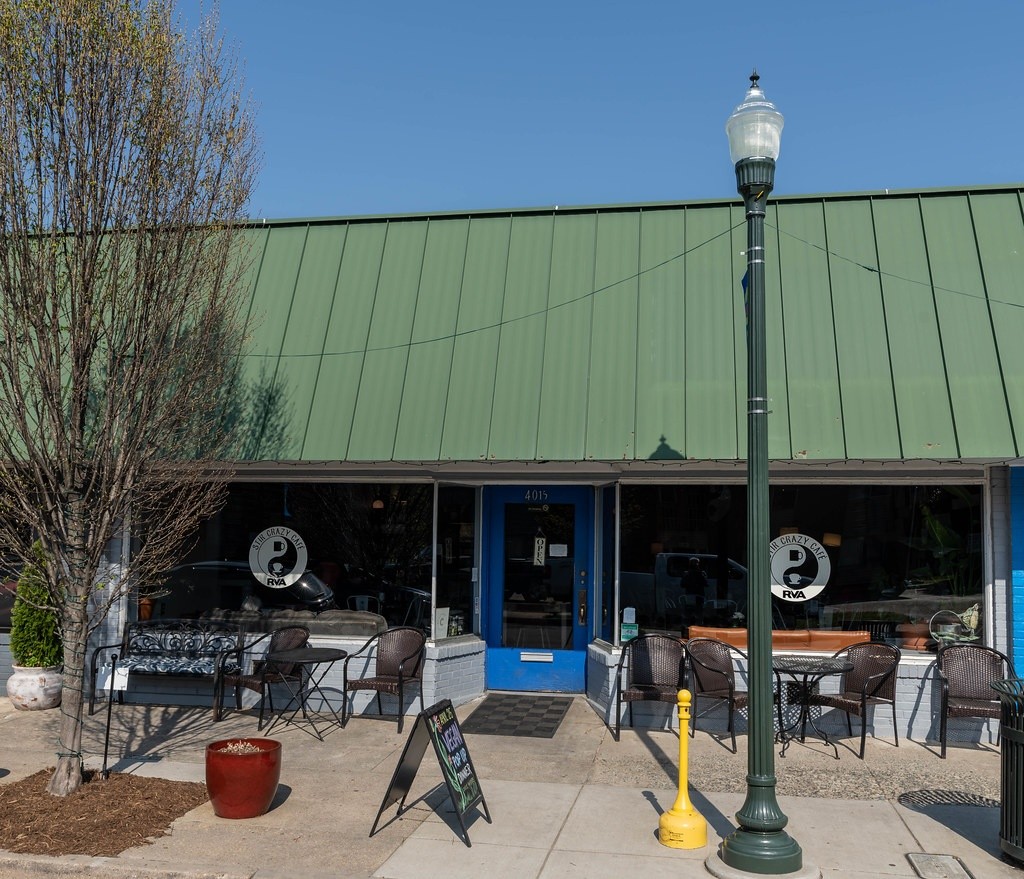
[770,655,855,759]
[265,648,349,741]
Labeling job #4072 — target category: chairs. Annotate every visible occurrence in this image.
[804,641,901,760]
[346,596,381,615]
[342,627,427,734]
[616,633,689,742]
[686,636,786,754]
[806,606,844,631]
[664,595,700,628]
[704,599,738,628]
[933,643,1023,759]
[217,625,310,731]
[402,595,430,628]
[771,604,788,630]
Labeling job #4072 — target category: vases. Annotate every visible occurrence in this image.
[205,738,281,820]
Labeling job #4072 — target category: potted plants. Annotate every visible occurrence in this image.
[6,538,63,710]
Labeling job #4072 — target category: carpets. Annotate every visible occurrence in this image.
[459,693,575,739]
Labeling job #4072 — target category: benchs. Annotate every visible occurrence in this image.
[199,608,389,636]
[88,620,245,722]
[688,626,870,652]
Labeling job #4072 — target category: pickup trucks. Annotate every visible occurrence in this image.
[619,552,748,629]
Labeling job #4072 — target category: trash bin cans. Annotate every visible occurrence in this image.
[990,679,1024,862]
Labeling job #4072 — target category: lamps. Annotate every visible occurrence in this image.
[373,487,384,509]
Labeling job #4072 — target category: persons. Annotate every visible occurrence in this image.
[681,557,707,626]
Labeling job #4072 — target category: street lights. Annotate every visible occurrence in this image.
[714,66,806,875]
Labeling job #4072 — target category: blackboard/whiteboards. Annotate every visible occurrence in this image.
[381,699,483,820]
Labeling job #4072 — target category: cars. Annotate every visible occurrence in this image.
[501,548,576,606]
[0,563,23,628]
[137,559,341,624]
[311,543,473,631]
